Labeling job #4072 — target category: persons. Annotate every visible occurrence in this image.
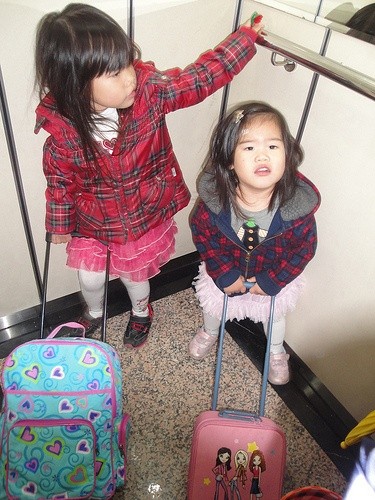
[187,100,321,385]
[34,4,265,348]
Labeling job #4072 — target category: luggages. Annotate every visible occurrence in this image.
[0,231,130,500]
[186,282,285,500]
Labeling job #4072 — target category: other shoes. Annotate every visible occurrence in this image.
[187,322,218,361]
[269,352,290,385]
[123,303,154,347]
[71,308,103,339]
